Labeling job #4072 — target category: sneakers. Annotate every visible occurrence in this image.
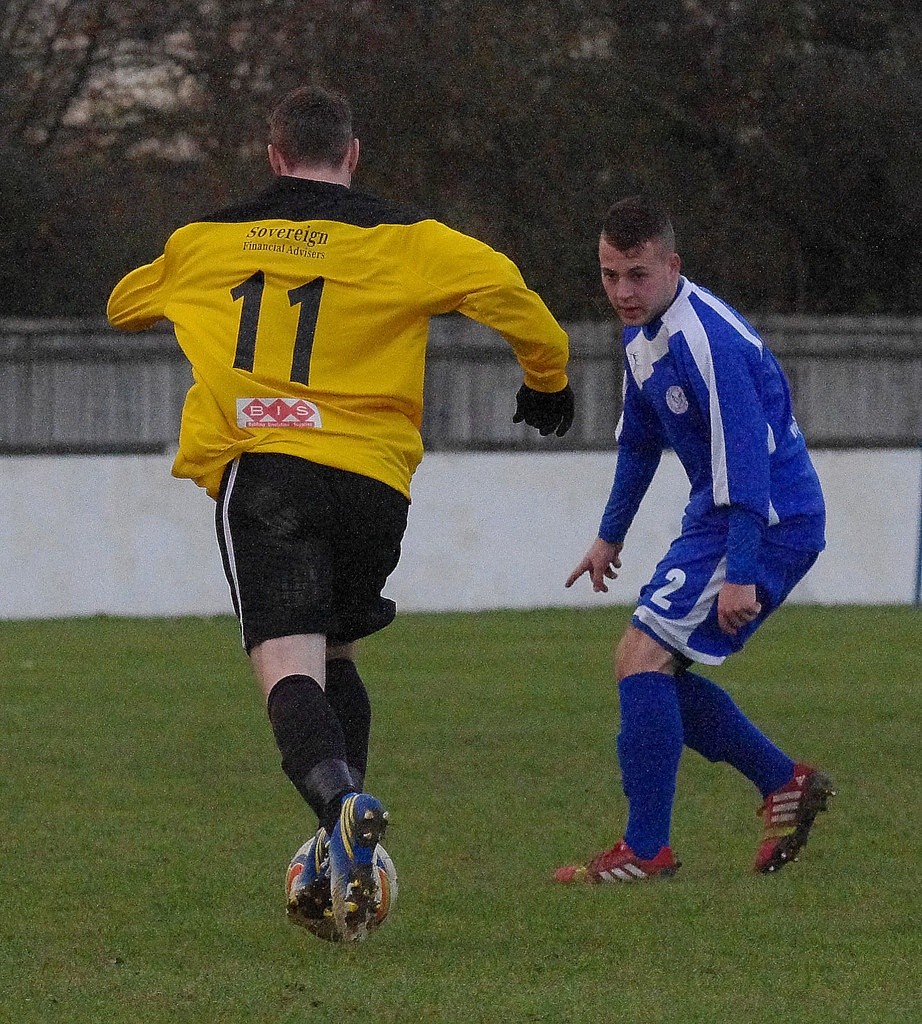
[286,814,334,918]
[552,834,681,886]
[754,761,837,873]
[328,791,390,942]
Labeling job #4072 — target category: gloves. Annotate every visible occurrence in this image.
[512,382,577,438]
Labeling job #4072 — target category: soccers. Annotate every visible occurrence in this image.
[284,834,399,943]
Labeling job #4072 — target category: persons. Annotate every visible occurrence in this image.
[554,196,836,885]
[106,88,574,939]
